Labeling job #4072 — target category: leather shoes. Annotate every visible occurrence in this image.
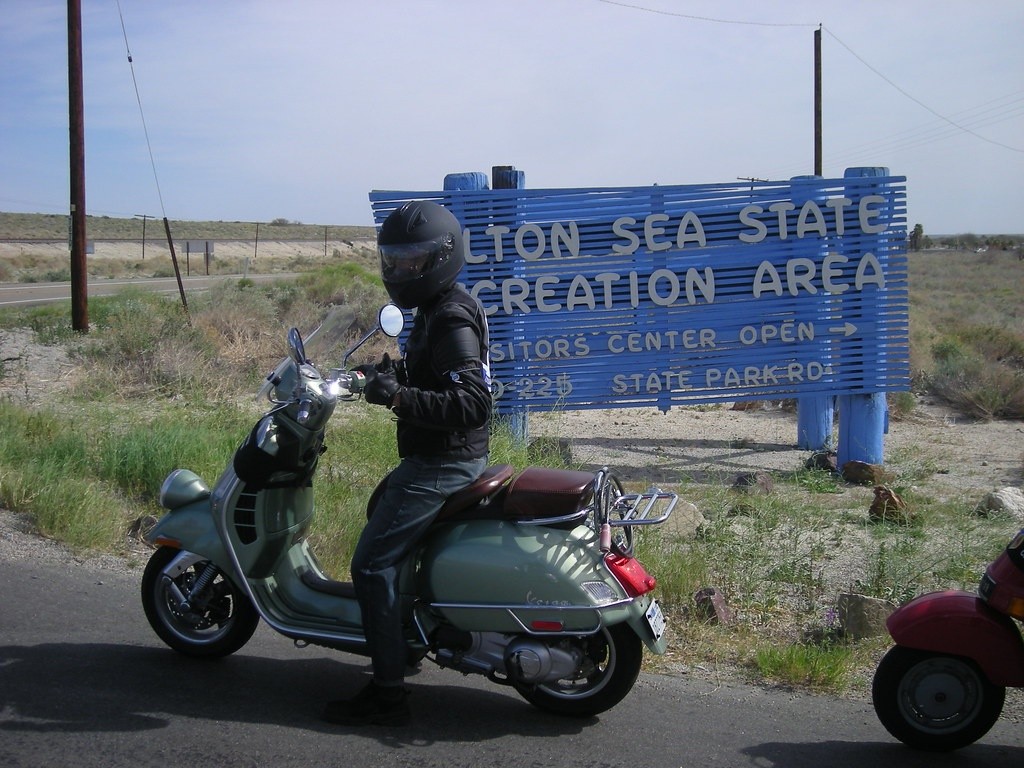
[324,679,411,727]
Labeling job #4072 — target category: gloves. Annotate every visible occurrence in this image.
[350,353,403,410]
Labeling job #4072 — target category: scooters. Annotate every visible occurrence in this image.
[140,303,681,719]
[871,526,1024,753]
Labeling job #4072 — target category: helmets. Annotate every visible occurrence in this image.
[378,200,465,309]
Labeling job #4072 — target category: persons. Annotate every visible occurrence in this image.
[319,201,491,727]
